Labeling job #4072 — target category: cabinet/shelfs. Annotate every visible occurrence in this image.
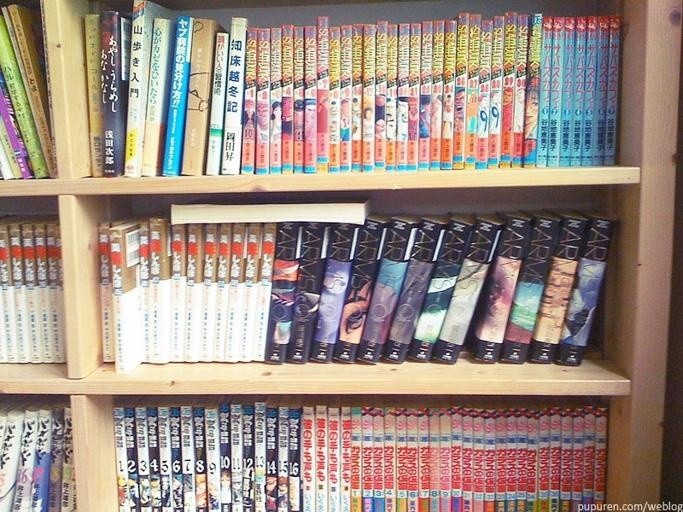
[0,1,681,512]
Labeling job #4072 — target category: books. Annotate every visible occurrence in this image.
[2,398,77,512]
[0,217,67,365]
[82,0,620,176]
[0,1,55,180]
[112,397,610,512]
[100,202,620,373]
